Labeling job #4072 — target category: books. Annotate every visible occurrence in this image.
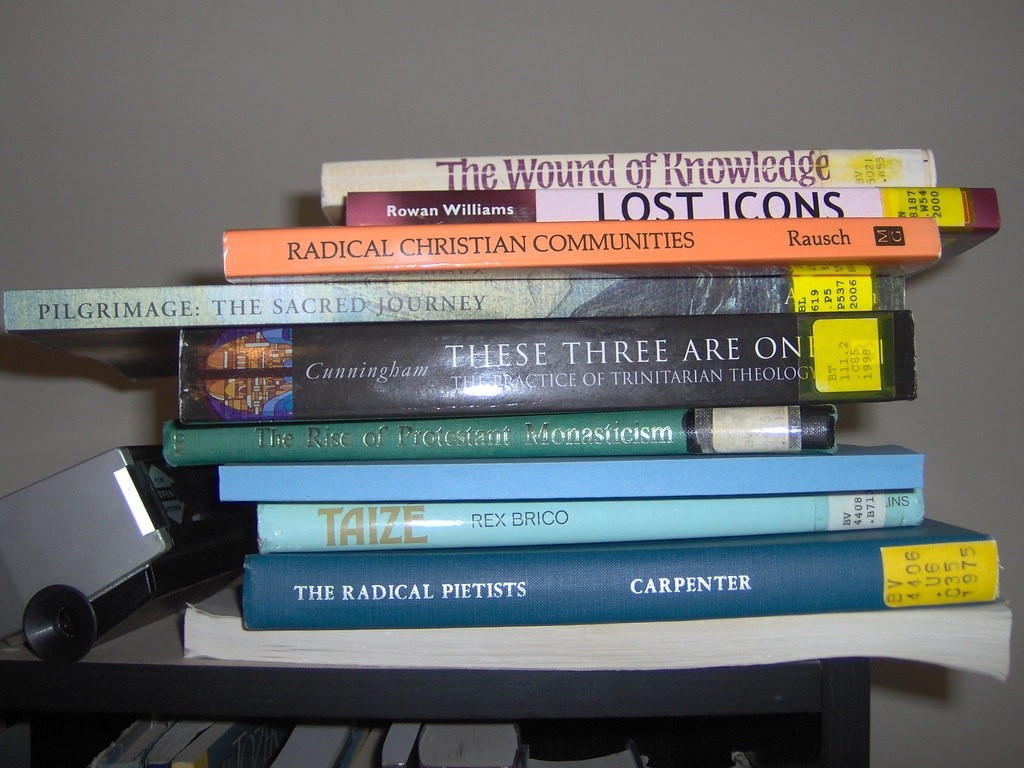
[4,145,1014,687]
[84,715,761,768]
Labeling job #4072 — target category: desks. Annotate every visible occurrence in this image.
[0,575,874,768]
